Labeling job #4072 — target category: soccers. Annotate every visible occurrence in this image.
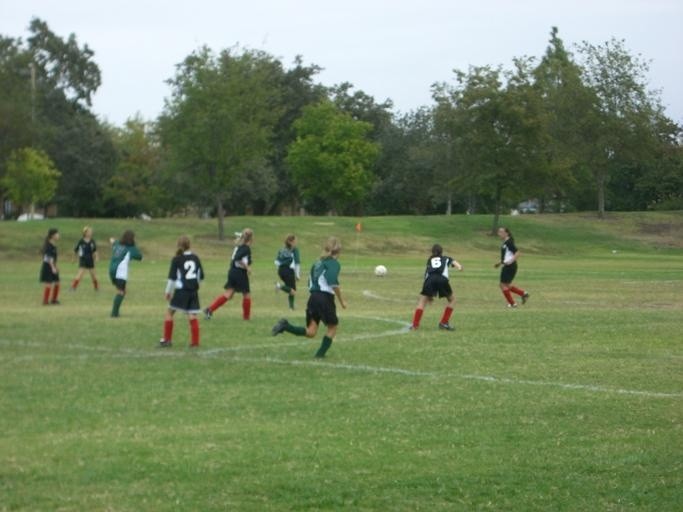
[373,265,387,277]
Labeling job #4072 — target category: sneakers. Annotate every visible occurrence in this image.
[159,338,171,348]
[270,318,287,337]
[438,322,455,330]
[522,292,529,304]
[276,281,280,296]
[508,304,519,308]
[203,309,212,321]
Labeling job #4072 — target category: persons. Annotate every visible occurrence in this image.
[492,226,531,308]
[203,227,255,320]
[38,228,61,307]
[270,236,347,358]
[108,230,143,318]
[158,236,206,347]
[407,242,463,331]
[69,224,102,291]
[274,234,301,311]
